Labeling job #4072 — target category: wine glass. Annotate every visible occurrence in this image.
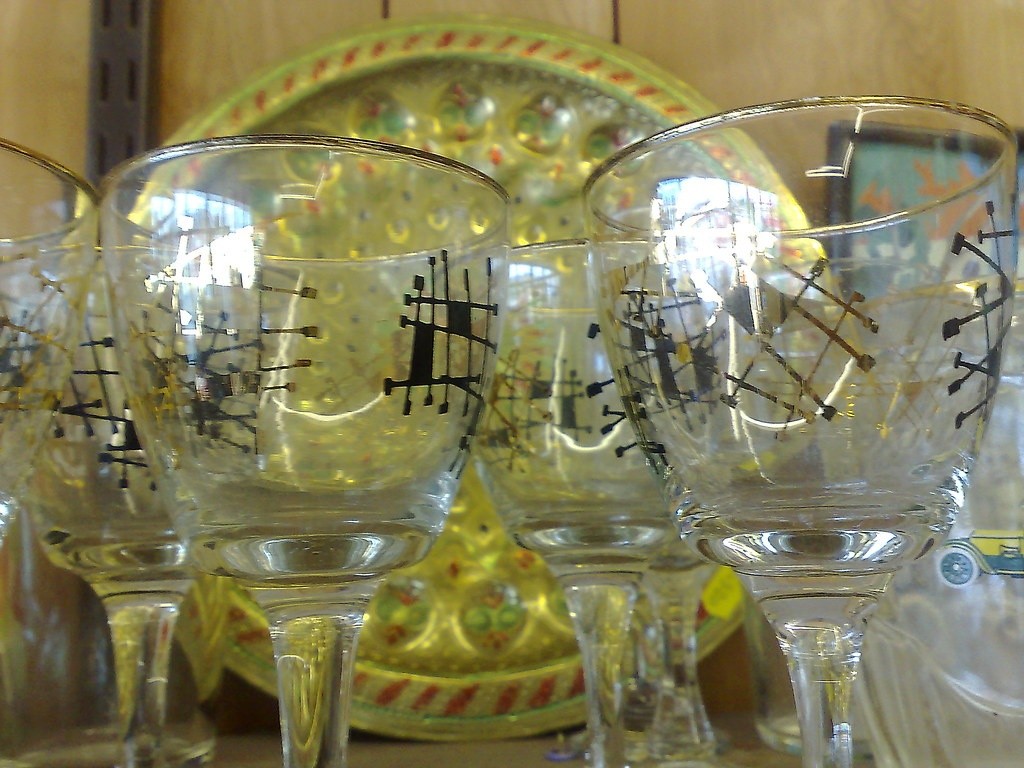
[0,93,1024,768]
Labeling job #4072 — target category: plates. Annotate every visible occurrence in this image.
[124,16,837,745]
